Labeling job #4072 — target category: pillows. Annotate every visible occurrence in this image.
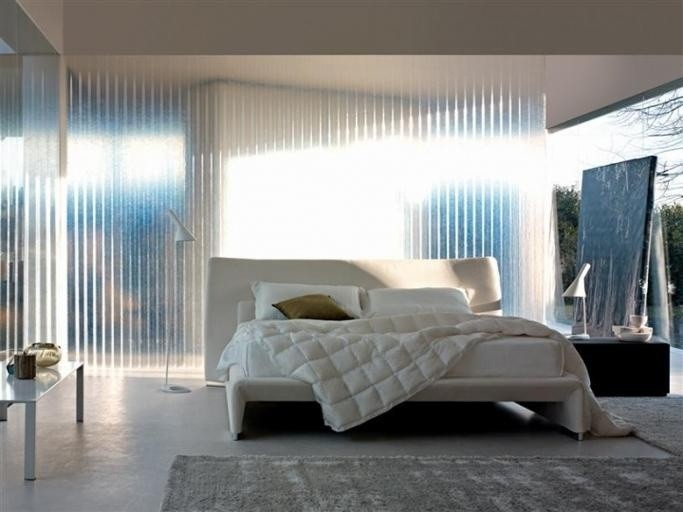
[250,280,475,321]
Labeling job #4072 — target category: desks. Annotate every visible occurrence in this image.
[562,335,670,397]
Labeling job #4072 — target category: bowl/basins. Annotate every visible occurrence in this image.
[618,332,652,342]
[629,315,648,328]
[610,325,653,341]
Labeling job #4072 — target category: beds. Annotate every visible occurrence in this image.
[225,315,590,442]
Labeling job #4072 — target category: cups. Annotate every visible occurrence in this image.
[14,351,36,380]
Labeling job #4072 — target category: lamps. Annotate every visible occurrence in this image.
[561,263,592,341]
[159,209,195,395]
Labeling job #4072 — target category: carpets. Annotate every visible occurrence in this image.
[161,457,683,512]
[596,393,683,457]
